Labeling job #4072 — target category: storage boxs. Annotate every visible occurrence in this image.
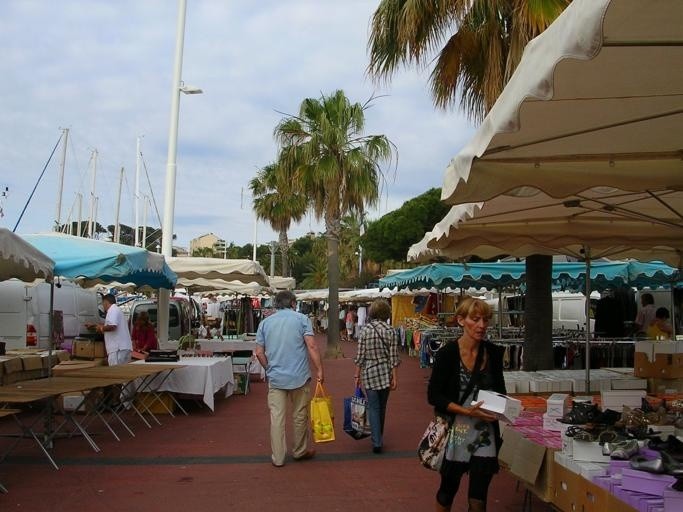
[470,341,683,512]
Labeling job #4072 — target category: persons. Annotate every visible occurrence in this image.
[427,298,522,511]
[256,290,324,469]
[351,299,397,455]
[127,309,159,359]
[84,294,133,412]
[307,298,369,342]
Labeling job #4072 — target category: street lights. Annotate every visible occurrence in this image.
[268,241,279,279]
[154,85,212,353]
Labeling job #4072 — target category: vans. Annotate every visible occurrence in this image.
[128,296,199,355]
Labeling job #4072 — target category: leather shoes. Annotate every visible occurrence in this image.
[610,439,640,460]
[302,447,316,457]
[602,441,630,456]
[628,455,665,474]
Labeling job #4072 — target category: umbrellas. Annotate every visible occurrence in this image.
[80,249,301,297]
[302,279,409,307]
[0,226,55,289]
[15,225,177,381]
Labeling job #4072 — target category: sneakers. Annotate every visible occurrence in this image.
[107,402,125,412]
[272,461,286,468]
[372,446,382,454]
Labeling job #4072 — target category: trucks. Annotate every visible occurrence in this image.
[551,286,672,335]
[0,277,107,354]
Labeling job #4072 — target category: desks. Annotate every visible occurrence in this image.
[0,350,255,495]
[169,334,266,382]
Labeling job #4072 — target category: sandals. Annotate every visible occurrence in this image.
[555,397,683,446]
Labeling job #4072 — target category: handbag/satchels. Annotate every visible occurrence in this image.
[308,379,336,446]
[345,321,353,330]
[342,384,373,442]
[416,415,452,472]
